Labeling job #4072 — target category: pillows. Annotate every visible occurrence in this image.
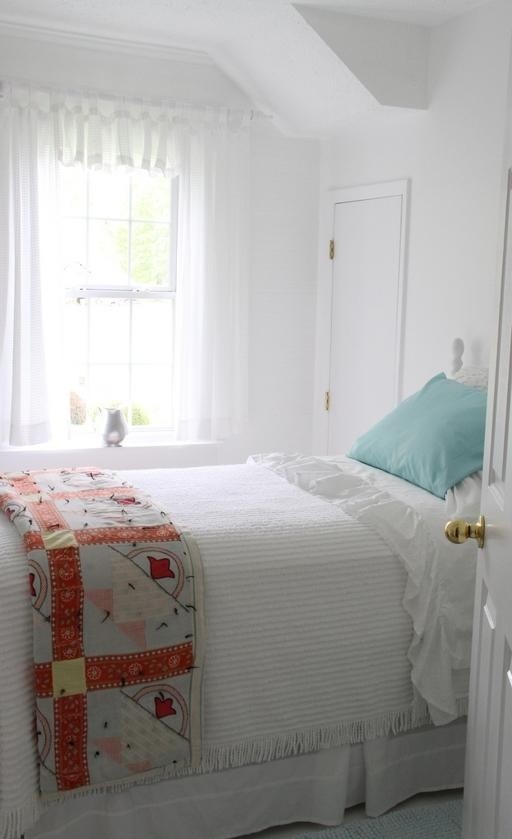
[345,371,488,501]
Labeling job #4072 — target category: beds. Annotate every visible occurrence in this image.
[0,337,489,839]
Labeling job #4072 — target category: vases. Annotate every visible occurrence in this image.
[102,407,126,446]
